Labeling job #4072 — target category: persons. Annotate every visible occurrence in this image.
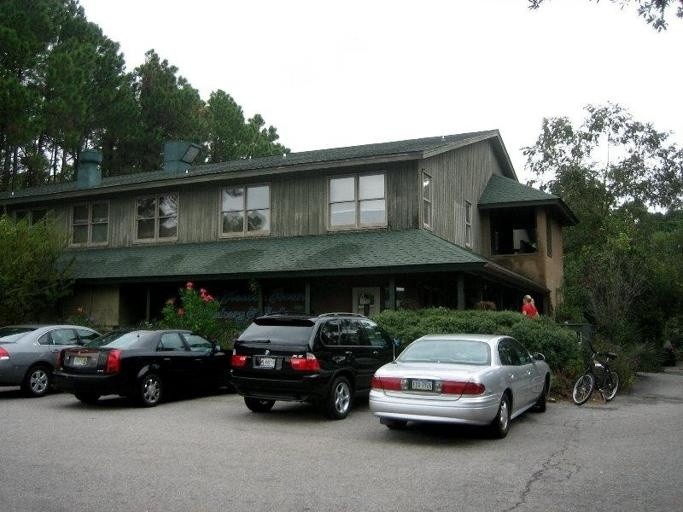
[522,295,541,317]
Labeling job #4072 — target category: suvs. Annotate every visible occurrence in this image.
[227,311,401,419]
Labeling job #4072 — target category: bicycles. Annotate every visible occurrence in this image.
[572,338,624,405]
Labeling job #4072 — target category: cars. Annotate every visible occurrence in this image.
[1,324,98,398]
[370,332,551,438]
[52,328,231,406]
